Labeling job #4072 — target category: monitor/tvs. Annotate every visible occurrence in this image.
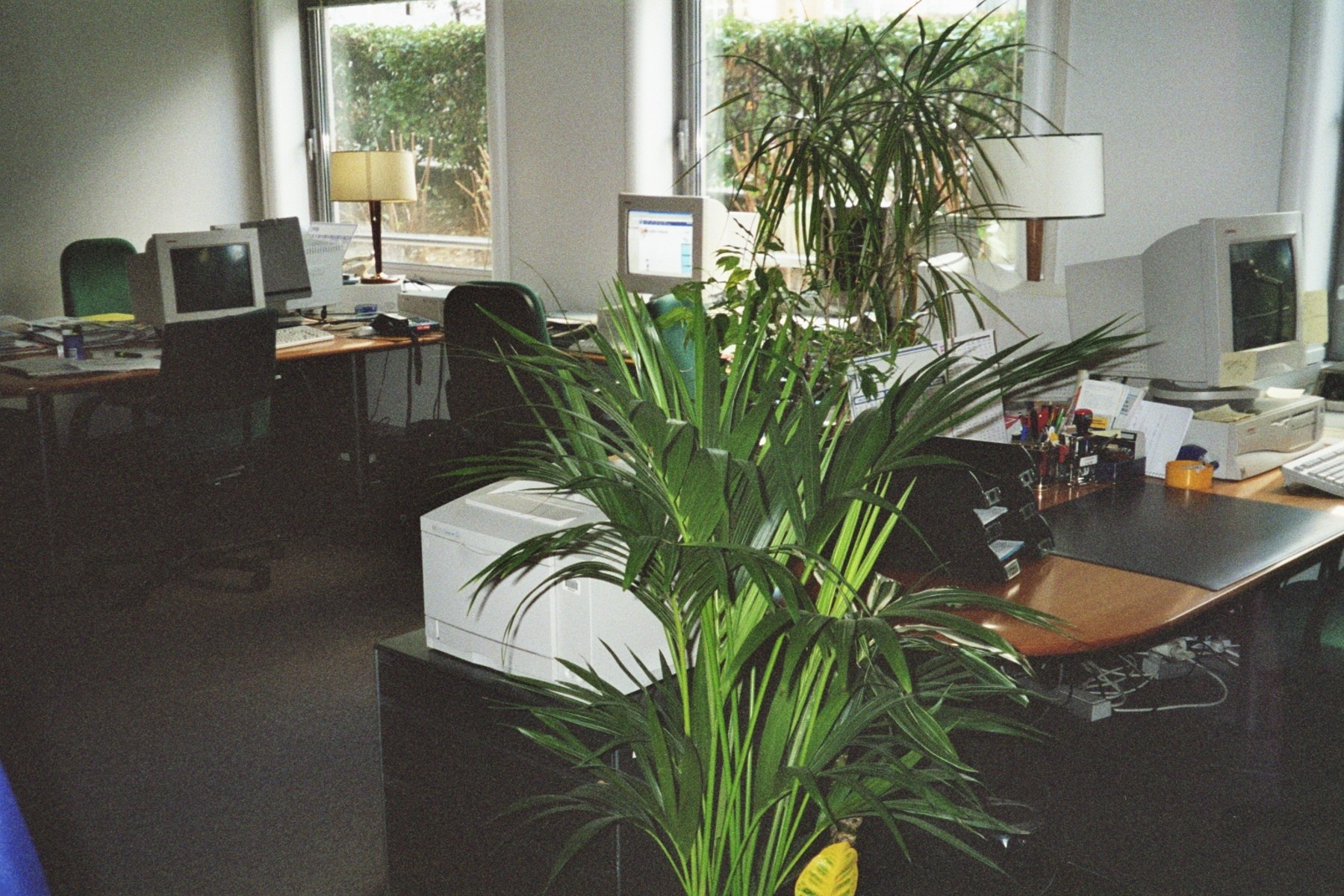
[617,192,739,304]
[127,227,265,327]
[1064,211,1306,400]
[818,204,915,311]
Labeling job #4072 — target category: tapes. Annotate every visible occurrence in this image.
[1165,460,1213,490]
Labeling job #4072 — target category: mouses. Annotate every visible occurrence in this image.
[349,326,373,337]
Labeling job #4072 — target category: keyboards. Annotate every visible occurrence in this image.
[1282,441,1344,497]
[569,340,630,358]
[274,326,335,349]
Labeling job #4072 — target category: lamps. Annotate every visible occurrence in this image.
[330,150,418,285]
[967,131,1108,286]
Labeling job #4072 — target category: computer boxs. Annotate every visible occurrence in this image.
[1139,386,1325,480]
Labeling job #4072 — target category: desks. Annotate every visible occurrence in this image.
[554,334,752,380]
[0,319,457,561]
[874,427,1344,896]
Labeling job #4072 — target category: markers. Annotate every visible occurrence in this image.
[114,352,143,358]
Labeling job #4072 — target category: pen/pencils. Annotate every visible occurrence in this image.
[1018,384,1082,443]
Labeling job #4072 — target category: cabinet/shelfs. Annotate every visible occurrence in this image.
[374,631,693,896]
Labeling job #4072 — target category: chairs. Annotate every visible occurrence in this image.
[72,308,289,596]
[61,237,138,323]
[443,282,643,483]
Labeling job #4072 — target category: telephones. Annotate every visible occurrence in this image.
[370,313,439,337]
